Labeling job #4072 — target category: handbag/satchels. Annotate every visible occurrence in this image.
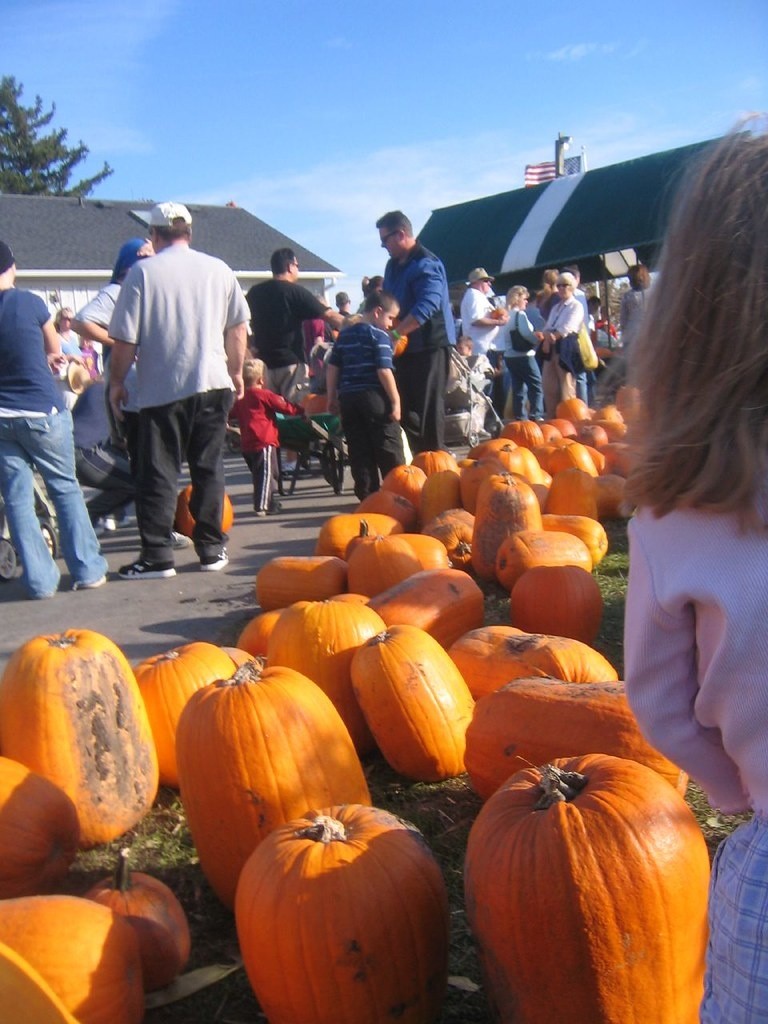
[509,311,534,352]
[578,321,598,370]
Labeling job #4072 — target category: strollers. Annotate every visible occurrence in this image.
[0,466,62,582]
[442,348,505,450]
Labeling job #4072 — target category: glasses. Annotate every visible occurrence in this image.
[556,283,570,287]
[380,230,401,243]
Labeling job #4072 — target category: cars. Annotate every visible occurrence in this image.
[594,329,627,388]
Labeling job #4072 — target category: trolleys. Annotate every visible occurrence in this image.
[225,411,349,495]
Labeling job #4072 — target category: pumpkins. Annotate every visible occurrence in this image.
[390,318,409,357]
[302,392,329,413]
[0,557,716,1024]
[492,308,508,320]
[318,384,643,640]
[175,483,233,536]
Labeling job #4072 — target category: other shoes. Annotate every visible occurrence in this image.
[282,461,299,480]
[29,584,57,599]
[254,502,282,512]
[93,526,105,536]
[77,574,107,589]
[486,424,498,433]
[256,507,280,516]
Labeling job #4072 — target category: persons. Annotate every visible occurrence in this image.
[0,240,110,600]
[326,290,407,502]
[71,237,194,550]
[593,107,768,1024]
[47,250,652,515]
[110,202,251,579]
[348,211,456,452]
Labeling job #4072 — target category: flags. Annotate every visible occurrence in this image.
[524,156,580,187]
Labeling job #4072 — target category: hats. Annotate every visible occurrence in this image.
[465,267,495,287]
[111,238,154,284]
[149,202,192,227]
[67,360,91,395]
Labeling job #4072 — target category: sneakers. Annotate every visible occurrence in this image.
[200,547,229,571]
[171,531,193,548]
[118,559,176,579]
[97,515,131,530]
